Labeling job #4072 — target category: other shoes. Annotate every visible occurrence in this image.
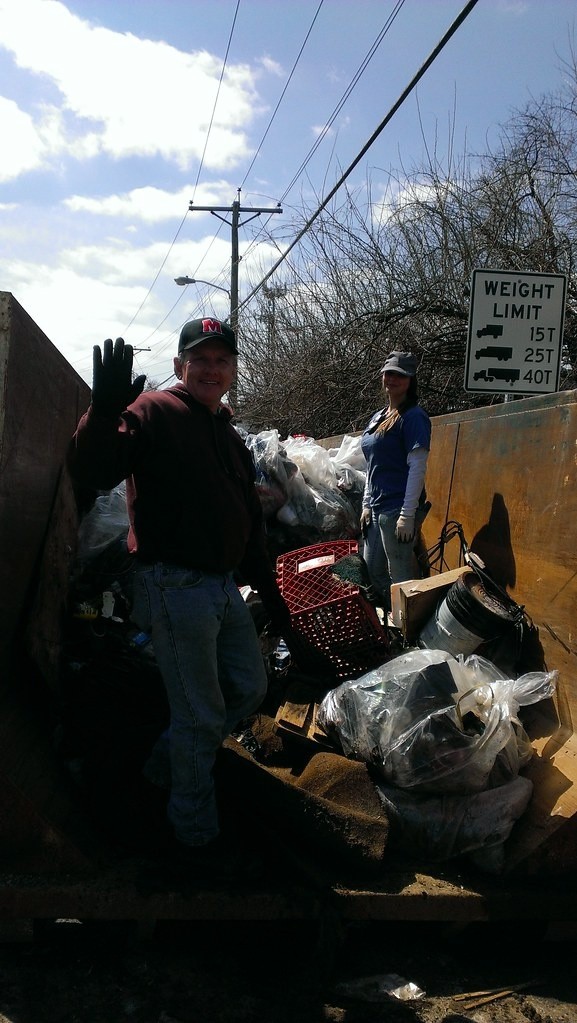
[174,848,264,883]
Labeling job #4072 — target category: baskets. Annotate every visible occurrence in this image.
[276,540,388,681]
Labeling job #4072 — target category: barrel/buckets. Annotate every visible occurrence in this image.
[419,584,490,665]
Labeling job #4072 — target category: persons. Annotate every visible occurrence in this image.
[70,318,268,893]
[358,352,432,600]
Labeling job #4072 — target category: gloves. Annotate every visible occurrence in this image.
[394,515,415,542]
[360,508,371,531]
[86,339,145,434]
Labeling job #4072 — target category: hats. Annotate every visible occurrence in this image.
[179,322,238,358]
[379,351,417,376]
